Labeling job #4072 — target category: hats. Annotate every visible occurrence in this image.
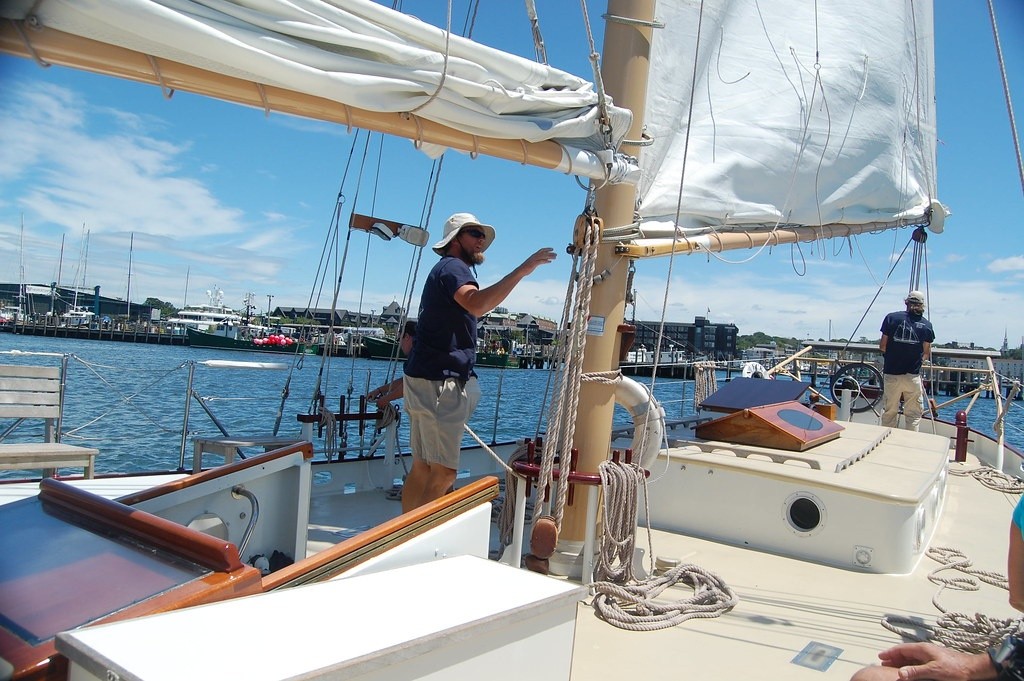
[907,290,924,303]
[432,213,496,254]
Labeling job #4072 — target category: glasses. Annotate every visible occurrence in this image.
[459,229,486,239]
[396,336,405,342]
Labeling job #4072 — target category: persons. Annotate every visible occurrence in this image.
[366,320,482,501]
[878,290,936,432]
[400,213,562,517]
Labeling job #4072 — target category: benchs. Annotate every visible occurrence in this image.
[193,435,306,476]
[0,357,100,481]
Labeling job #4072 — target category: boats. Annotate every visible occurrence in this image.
[0,210,891,378]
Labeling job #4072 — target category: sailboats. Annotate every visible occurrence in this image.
[0,1,1024,680]
